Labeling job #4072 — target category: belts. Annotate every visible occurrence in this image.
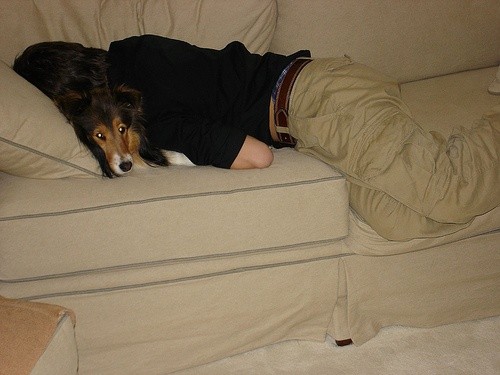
[276,59,312,145]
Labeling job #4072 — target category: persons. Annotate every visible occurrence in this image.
[13,34,500,242]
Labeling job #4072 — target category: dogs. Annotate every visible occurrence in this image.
[51,87,197,179]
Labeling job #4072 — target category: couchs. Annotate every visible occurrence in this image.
[0,0,500,375]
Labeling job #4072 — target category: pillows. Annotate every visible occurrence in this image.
[0,56,104,180]
